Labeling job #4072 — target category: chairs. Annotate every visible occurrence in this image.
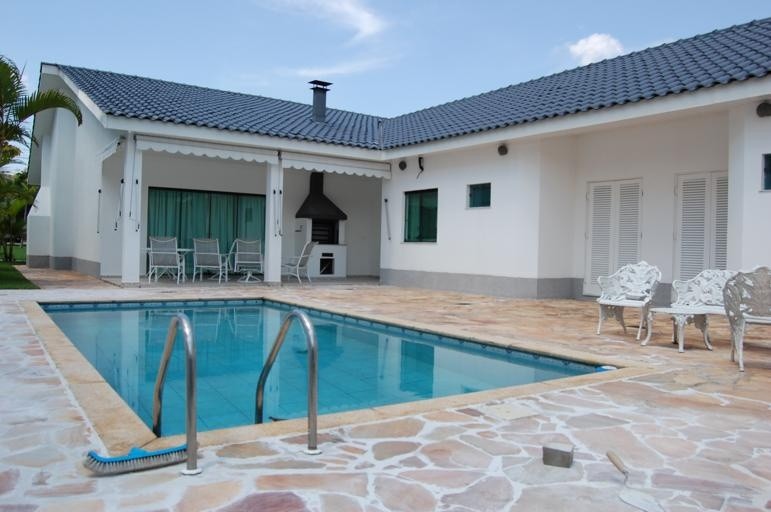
[236,239,264,284]
[148,236,186,285]
[280,241,319,285]
[669,269,749,345]
[723,266,771,372]
[192,238,235,285]
[596,260,663,341]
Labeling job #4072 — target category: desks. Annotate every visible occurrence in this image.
[640,307,714,353]
[144,248,195,282]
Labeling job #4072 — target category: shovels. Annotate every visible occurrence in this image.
[606,450,665,512]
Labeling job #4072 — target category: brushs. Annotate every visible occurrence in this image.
[81,441,199,476]
